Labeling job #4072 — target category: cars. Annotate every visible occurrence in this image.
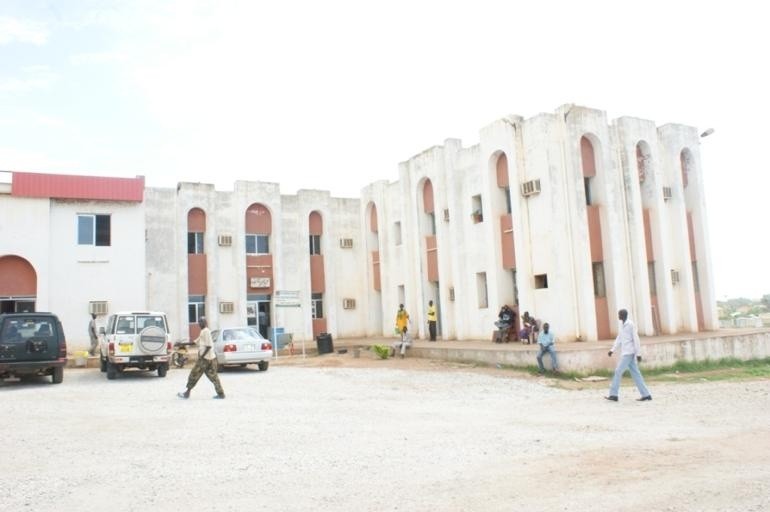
[198,327,273,371]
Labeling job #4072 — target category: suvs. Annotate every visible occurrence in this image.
[100,312,174,379]
[0,312,67,383]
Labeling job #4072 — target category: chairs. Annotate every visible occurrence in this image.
[4,322,51,342]
[117,318,156,333]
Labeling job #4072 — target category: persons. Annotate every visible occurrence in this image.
[393,326,414,356]
[86,312,100,358]
[495,305,540,344]
[601,307,653,402]
[535,322,562,376]
[395,302,411,337]
[173,315,226,399]
[425,299,439,341]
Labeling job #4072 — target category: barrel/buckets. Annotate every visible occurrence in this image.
[316,332,333,354]
[353,349,360,357]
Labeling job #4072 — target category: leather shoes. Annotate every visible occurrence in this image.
[636,395,652,401]
[605,395,618,401]
